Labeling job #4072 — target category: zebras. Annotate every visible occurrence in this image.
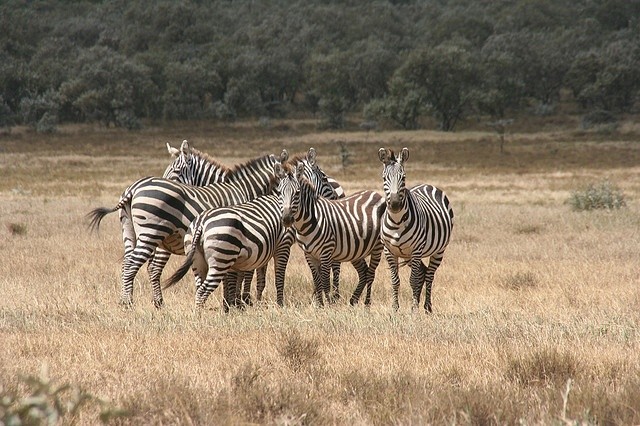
[84,145,295,315]
[272,159,387,315]
[378,145,456,319]
